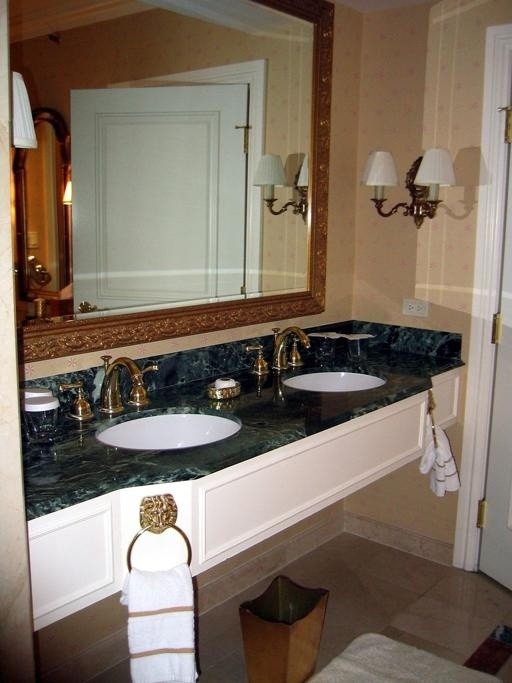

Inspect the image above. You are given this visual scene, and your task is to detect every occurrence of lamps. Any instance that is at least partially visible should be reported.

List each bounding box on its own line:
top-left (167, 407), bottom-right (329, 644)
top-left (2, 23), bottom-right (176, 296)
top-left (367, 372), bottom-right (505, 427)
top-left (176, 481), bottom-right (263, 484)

top-left (252, 153), bottom-right (310, 223)
top-left (10, 71), bottom-right (40, 151)
top-left (360, 147), bottom-right (457, 230)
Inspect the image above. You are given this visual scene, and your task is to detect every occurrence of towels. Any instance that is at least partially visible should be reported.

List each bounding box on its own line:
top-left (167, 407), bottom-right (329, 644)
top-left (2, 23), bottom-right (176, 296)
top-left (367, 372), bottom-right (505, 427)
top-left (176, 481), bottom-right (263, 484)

top-left (418, 425), bottom-right (462, 498)
top-left (119, 562), bottom-right (202, 682)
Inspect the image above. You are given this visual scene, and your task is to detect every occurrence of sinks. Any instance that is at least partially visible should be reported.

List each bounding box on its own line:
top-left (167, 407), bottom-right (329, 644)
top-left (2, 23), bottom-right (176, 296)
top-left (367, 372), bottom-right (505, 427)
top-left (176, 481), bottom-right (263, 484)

top-left (281, 368), bottom-right (388, 393)
top-left (95, 406), bottom-right (242, 450)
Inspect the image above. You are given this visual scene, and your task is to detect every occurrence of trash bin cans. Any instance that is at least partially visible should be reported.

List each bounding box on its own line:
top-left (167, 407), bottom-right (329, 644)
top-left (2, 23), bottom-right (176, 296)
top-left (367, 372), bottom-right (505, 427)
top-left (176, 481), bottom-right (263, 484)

top-left (240, 575), bottom-right (329, 683)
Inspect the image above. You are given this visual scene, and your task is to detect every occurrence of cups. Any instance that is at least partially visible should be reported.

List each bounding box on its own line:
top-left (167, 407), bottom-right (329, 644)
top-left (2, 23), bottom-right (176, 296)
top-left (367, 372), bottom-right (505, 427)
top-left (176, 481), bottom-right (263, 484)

top-left (306, 332), bottom-right (376, 365)
top-left (24, 387), bottom-right (60, 444)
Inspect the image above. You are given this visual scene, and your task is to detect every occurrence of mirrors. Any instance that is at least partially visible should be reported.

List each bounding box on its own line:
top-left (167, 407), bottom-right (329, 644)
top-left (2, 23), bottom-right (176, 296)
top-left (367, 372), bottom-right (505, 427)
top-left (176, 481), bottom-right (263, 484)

top-left (12, 107), bottom-right (72, 306)
top-left (7, 0), bottom-right (337, 367)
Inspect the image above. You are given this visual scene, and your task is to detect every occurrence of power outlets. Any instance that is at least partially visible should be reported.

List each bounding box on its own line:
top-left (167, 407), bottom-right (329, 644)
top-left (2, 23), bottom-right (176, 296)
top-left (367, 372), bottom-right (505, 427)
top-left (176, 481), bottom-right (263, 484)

top-left (402, 297), bottom-right (429, 318)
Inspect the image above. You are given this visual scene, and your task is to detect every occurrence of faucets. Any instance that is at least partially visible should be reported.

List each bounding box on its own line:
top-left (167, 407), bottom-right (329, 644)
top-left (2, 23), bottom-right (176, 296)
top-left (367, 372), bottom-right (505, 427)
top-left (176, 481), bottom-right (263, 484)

top-left (271, 327), bottom-right (311, 368)
top-left (100, 358), bottom-right (152, 413)
top-left (274, 371), bottom-right (292, 408)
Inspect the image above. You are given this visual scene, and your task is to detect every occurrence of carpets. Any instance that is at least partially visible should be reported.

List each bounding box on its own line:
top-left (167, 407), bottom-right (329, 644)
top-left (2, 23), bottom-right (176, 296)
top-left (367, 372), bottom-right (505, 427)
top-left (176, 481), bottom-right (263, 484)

top-left (309, 632), bottom-right (502, 683)
